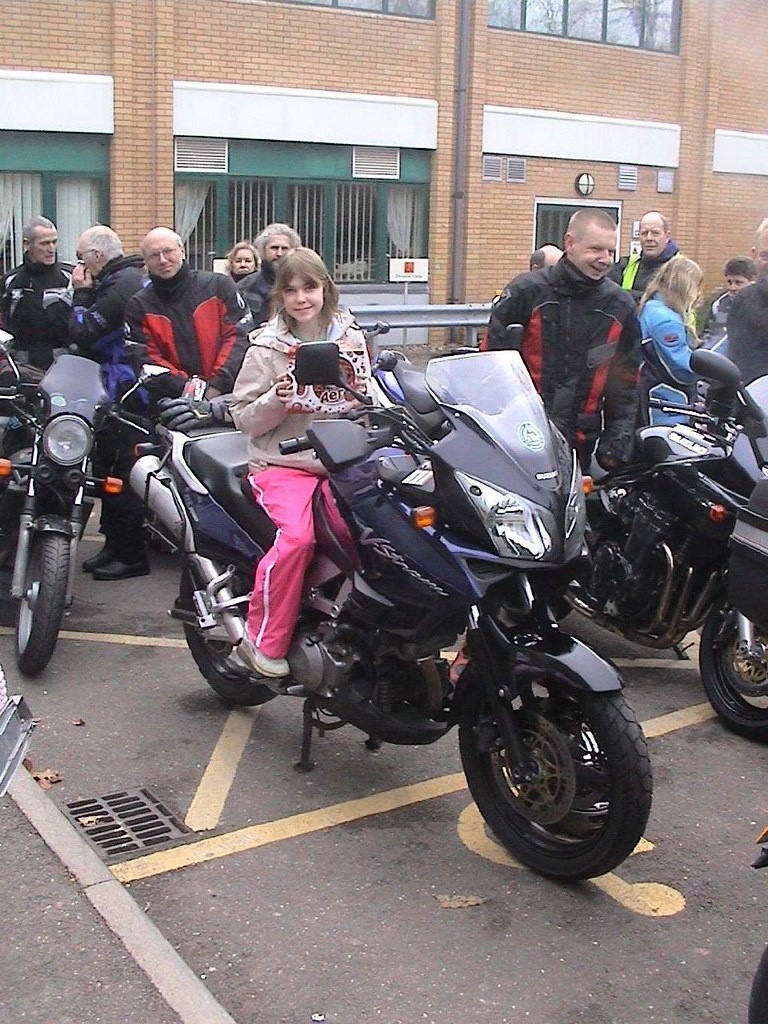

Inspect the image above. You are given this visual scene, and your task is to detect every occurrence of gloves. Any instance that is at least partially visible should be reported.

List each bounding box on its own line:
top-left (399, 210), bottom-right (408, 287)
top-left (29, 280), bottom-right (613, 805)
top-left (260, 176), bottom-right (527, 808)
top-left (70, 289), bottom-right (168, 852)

top-left (159, 395), bottom-right (233, 431)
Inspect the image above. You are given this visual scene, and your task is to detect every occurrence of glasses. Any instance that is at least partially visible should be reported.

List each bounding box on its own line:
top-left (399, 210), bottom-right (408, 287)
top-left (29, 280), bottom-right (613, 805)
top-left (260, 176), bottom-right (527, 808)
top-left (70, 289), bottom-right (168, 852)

top-left (145, 245), bottom-right (181, 262)
top-left (76, 249), bottom-right (104, 264)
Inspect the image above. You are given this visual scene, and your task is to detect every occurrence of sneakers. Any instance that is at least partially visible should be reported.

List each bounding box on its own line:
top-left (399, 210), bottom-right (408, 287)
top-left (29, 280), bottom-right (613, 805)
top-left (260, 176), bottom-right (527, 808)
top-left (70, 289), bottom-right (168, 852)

top-left (240, 622), bottom-right (290, 678)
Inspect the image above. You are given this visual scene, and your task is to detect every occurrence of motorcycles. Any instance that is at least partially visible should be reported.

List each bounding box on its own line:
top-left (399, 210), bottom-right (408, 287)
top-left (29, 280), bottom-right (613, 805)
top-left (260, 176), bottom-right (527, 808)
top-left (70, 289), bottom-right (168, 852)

top-left (554, 348), bottom-right (767, 736)
top-left (1, 329), bottom-right (170, 678)
top-left (131, 325), bottom-right (656, 884)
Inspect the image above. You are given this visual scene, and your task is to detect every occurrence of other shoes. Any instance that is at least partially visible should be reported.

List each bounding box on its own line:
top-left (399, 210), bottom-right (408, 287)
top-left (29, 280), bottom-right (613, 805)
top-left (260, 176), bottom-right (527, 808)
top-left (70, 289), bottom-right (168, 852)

top-left (92, 558), bottom-right (150, 581)
top-left (82, 545), bottom-right (116, 570)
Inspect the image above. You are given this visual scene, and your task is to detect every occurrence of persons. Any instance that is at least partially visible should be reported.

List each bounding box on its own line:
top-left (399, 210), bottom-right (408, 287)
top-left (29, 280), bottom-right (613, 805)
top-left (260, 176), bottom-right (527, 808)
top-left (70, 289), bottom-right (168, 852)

top-left (229, 247), bottom-right (379, 676)
top-left (530, 210), bottom-right (768, 476)
top-left (0, 216), bottom-right (303, 581)
top-left (450, 208), bottom-right (643, 683)
